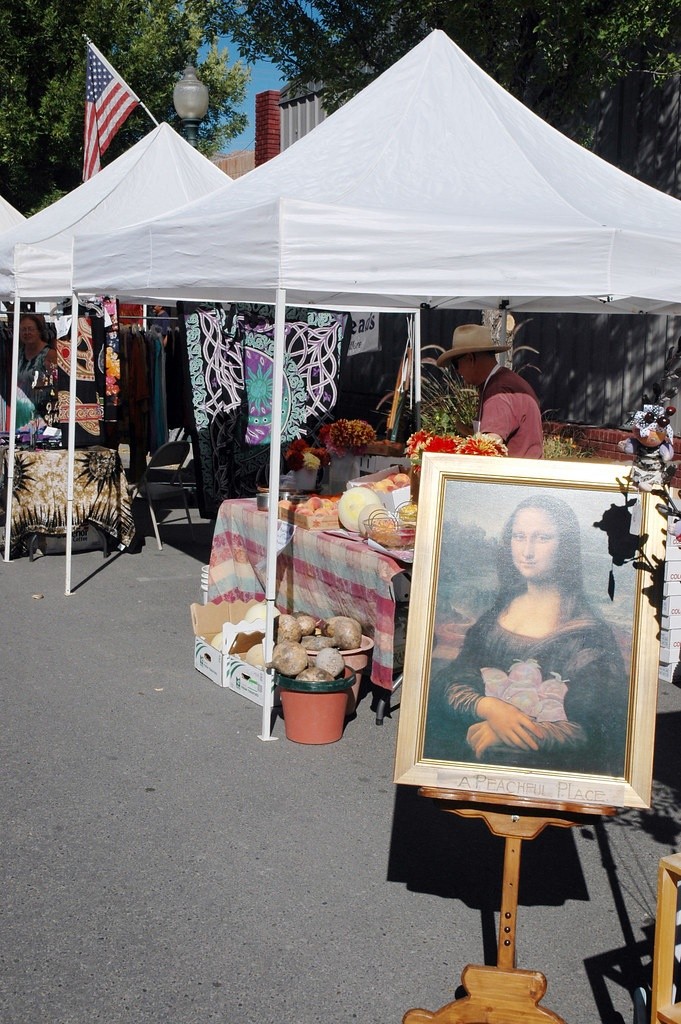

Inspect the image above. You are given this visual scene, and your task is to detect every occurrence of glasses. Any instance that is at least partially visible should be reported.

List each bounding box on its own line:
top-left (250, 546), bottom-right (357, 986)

top-left (449, 354), bottom-right (466, 371)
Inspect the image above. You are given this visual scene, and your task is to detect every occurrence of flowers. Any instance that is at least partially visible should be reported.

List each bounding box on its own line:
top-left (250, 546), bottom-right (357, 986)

top-left (404, 430), bottom-right (508, 477)
top-left (318, 418), bottom-right (377, 459)
top-left (284, 439), bottom-right (330, 472)
top-left (372, 439), bottom-right (402, 455)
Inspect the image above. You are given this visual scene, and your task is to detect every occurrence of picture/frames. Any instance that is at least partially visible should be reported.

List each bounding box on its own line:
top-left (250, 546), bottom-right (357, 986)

top-left (392, 449), bottom-right (670, 808)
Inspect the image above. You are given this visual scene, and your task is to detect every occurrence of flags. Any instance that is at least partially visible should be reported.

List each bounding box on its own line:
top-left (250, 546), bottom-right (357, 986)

top-left (82, 43), bottom-right (138, 183)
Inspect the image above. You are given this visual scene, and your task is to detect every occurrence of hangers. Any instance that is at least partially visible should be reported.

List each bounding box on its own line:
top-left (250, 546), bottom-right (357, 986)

top-left (50, 289), bottom-right (103, 318)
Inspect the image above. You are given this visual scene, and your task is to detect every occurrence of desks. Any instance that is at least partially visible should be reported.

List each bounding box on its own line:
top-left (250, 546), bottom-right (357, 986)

top-left (207, 498), bottom-right (410, 689)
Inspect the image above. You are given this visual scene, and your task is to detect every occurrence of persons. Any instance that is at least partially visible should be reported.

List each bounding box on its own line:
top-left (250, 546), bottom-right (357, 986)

top-left (103, 297), bottom-right (115, 324)
top-left (18, 314), bottom-right (57, 417)
top-left (152, 305), bottom-right (170, 347)
top-left (436, 323), bottom-right (544, 460)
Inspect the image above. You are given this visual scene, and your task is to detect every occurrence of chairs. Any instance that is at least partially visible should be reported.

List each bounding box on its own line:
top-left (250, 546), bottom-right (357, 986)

top-left (128, 440), bottom-right (196, 551)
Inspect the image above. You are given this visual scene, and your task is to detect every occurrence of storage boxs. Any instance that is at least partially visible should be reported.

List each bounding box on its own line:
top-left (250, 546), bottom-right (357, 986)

top-left (358, 455), bottom-right (410, 473)
top-left (278, 506), bottom-right (341, 531)
top-left (346, 464), bottom-right (410, 516)
top-left (190, 599), bottom-right (282, 708)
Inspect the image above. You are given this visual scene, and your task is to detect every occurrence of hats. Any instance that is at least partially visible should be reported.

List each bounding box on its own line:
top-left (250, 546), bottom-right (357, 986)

top-left (434, 324), bottom-right (511, 367)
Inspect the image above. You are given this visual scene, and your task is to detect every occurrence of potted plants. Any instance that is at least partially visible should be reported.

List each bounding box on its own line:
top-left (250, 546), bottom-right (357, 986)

top-left (280, 467), bottom-right (318, 490)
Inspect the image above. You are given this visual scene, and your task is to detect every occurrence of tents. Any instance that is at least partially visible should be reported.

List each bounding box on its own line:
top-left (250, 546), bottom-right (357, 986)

top-left (64, 28), bottom-right (681, 741)
top-left (0, 121), bottom-right (235, 562)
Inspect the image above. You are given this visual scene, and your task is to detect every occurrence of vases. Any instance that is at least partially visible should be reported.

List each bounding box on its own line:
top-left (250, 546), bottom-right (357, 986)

top-left (329, 456), bottom-right (360, 494)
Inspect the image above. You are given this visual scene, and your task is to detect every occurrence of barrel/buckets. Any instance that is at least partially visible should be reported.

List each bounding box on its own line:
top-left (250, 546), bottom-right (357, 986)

top-left (200, 565), bottom-right (210, 605)
top-left (273, 663), bottom-right (357, 745)
top-left (305, 628), bottom-right (376, 716)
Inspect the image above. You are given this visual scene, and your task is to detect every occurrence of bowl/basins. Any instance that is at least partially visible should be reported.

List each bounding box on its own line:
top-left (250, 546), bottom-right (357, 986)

top-left (338, 487), bottom-right (380, 532)
top-left (362, 517), bottom-right (417, 547)
top-left (358, 504), bottom-right (388, 537)
top-left (279, 491), bottom-right (294, 502)
top-left (287, 495), bottom-right (310, 504)
top-left (256, 492), bottom-right (281, 510)
